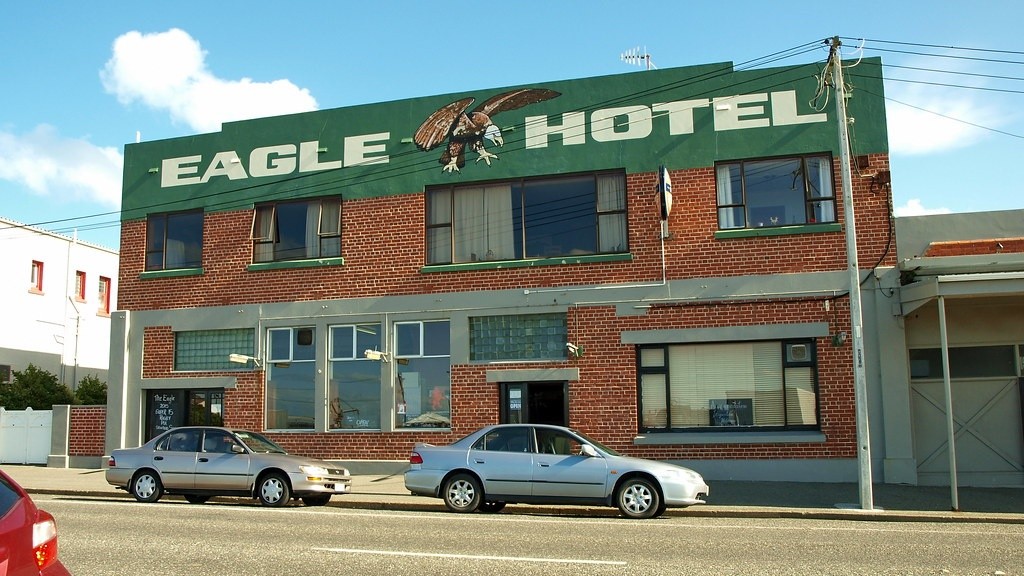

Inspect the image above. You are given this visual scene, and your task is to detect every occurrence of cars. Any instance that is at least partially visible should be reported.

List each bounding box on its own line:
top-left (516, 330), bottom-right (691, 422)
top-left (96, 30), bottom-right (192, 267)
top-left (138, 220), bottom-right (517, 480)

top-left (0, 470), bottom-right (71, 576)
top-left (106, 425), bottom-right (352, 508)
top-left (403, 423), bottom-right (708, 519)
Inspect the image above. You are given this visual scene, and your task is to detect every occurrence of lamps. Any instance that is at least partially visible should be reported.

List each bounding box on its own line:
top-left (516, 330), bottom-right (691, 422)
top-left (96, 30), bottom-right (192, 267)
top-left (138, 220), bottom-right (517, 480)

top-left (566, 342), bottom-right (579, 358)
top-left (229, 353), bottom-right (260, 368)
top-left (365, 349), bottom-right (388, 364)
